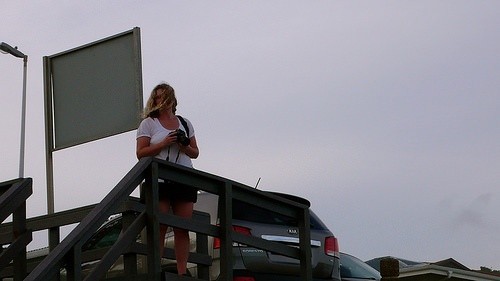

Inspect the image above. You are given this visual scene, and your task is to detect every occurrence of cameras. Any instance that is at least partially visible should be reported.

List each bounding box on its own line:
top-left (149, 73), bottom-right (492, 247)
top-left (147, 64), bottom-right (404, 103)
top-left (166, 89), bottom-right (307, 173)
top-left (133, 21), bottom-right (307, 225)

top-left (168, 129), bottom-right (191, 146)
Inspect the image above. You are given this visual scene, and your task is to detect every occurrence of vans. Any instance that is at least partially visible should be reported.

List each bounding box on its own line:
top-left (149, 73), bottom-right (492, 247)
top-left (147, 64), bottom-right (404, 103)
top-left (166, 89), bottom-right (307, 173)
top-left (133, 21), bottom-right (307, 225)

top-left (61, 191), bottom-right (340, 281)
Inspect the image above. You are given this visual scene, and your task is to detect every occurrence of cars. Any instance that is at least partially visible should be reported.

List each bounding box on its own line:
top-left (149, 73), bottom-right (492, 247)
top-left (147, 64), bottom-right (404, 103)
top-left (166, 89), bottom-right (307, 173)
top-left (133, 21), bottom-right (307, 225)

top-left (341, 251), bottom-right (432, 281)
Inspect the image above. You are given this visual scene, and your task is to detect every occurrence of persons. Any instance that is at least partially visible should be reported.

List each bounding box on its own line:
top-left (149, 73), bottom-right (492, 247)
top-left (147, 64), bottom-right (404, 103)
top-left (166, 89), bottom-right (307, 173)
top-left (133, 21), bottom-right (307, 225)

top-left (135, 84), bottom-right (199, 277)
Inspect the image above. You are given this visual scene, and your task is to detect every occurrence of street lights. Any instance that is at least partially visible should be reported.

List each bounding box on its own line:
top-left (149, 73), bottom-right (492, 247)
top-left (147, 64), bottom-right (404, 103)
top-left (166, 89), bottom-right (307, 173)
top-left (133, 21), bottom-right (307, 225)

top-left (1, 41), bottom-right (28, 178)
